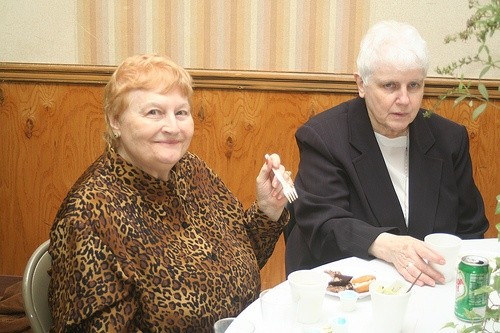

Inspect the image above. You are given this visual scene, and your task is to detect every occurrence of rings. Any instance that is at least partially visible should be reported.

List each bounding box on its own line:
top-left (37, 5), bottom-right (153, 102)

top-left (285, 170), bottom-right (291, 177)
top-left (404, 262), bottom-right (413, 270)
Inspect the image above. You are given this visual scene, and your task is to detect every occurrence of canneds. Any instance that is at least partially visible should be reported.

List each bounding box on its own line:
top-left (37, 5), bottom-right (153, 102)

top-left (454, 255), bottom-right (490, 324)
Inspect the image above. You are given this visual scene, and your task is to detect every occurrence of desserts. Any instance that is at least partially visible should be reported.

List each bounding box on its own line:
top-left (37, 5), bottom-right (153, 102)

top-left (326, 273), bottom-right (354, 293)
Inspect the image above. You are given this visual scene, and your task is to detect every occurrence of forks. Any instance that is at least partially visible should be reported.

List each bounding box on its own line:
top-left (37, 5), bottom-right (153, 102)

top-left (265, 154), bottom-right (298, 204)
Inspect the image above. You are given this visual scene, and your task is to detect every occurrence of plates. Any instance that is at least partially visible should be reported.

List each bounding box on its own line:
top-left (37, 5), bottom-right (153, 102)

top-left (321, 269), bottom-right (379, 298)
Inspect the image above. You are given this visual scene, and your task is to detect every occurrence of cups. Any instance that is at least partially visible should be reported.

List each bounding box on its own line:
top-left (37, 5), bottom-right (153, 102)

top-left (259, 288), bottom-right (301, 333)
top-left (213, 317), bottom-right (256, 333)
top-left (424, 234), bottom-right (465, 282)
top-left (340, 291), bottom-right (359, 313)
top-left (287, 268), bottom-right (332, 321)
top-left (368, 278), bottom-right (412, 330)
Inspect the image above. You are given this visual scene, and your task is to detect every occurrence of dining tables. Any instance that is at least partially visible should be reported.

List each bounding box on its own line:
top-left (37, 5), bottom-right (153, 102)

top-left (223, 239), bottom-right (500, 333)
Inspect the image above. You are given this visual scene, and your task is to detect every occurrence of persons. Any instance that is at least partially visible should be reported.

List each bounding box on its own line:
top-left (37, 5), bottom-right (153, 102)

top-left (47, 55), bottom-right (292, 333)
top-left (293, 21), bottom-right (490, 287)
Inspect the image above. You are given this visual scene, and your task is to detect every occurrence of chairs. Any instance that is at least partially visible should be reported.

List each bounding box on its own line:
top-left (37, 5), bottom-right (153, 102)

top-left (22, 239), bottom-right (52, 333)
